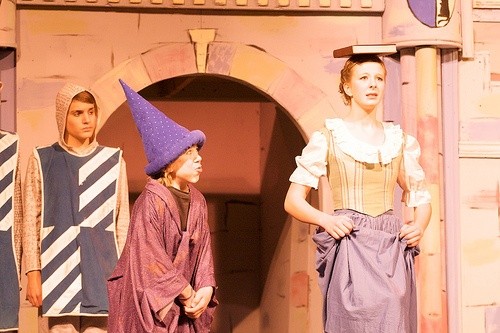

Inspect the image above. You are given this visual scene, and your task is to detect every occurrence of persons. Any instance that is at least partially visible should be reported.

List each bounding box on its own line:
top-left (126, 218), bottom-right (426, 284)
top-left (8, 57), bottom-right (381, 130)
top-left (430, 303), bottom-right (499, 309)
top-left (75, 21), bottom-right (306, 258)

top-left (21, 84), bottom-right (130, 333)
top-left (108, 79), bottom-right (219, 333)
top-left (284, 53), bottom-right (432, 333)
top-left (0, 128), bottom-right (23, 333)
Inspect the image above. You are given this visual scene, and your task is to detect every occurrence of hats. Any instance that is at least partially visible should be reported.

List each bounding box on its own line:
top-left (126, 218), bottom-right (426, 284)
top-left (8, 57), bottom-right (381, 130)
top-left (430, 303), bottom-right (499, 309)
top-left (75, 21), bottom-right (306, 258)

top-left (119, 77), bottom-right (205, 176)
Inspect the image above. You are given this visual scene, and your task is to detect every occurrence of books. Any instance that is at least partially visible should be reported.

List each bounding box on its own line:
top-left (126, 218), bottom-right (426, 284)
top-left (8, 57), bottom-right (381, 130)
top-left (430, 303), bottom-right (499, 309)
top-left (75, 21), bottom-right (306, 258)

top-left (333, 44), bottom-right (397, 58)
